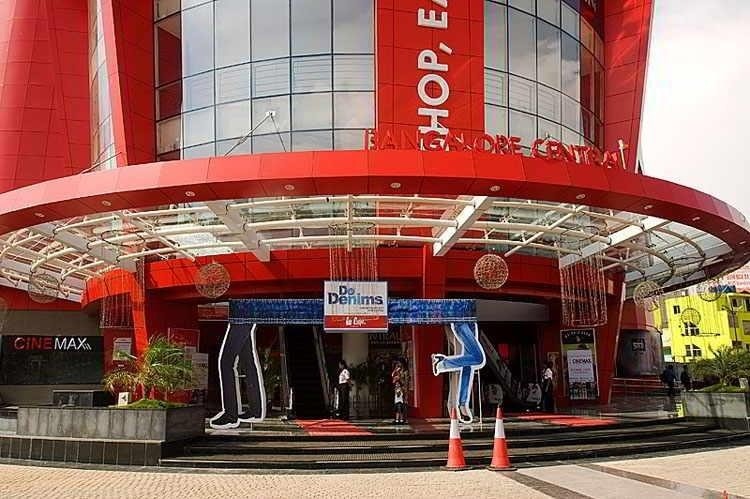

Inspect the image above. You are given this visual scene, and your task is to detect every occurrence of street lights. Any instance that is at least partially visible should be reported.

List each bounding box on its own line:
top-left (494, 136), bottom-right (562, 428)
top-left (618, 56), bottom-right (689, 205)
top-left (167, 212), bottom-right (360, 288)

top-left (721, 299), bottom-right (744, 353)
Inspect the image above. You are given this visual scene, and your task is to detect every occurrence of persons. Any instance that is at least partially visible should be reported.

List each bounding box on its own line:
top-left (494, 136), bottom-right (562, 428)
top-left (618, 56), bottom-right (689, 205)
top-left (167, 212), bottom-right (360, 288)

top-left (334, 359), bottom-right (354, 419)
top-left (539, 361), bottom-right (557, 412)
top-left (394, 380), bottom-right (406, 425)
top-left (680, 363), bottom-right (692, 392)
top-left (208, 323), bottom-right (267, 430)
top-left (664, 364), bottom-right (677, 397)
top-left (431, 320), bottom-right (488, 426)
top-left (391, 357), bottom-right (411, 423)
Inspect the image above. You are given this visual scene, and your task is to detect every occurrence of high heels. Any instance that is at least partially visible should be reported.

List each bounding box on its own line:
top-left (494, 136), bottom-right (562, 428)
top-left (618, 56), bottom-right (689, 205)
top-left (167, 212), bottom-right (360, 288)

top-left (458, 405), bottom-right (471, 423)
top-left (432, 354), bottom-right (445, 376)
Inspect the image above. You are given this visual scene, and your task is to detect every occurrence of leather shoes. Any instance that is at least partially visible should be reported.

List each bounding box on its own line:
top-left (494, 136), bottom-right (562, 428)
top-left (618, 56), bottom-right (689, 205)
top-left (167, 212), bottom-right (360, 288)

top-left (238, 409), bottom-right (262, 420)
top-left (210, 412), bottom-right (238, 425)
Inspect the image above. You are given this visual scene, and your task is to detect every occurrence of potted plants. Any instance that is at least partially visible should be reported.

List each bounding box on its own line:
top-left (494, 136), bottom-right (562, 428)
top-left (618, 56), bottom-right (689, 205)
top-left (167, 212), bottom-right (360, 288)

top-left (688, 342), bottom-right (749, 390)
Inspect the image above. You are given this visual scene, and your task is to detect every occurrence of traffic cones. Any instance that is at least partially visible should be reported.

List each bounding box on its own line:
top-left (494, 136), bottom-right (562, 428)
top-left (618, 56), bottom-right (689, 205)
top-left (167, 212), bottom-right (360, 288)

top-left (485, 403), bottom-right (518, 472)
top-left (439, 408), bottom-right (474, 471)
